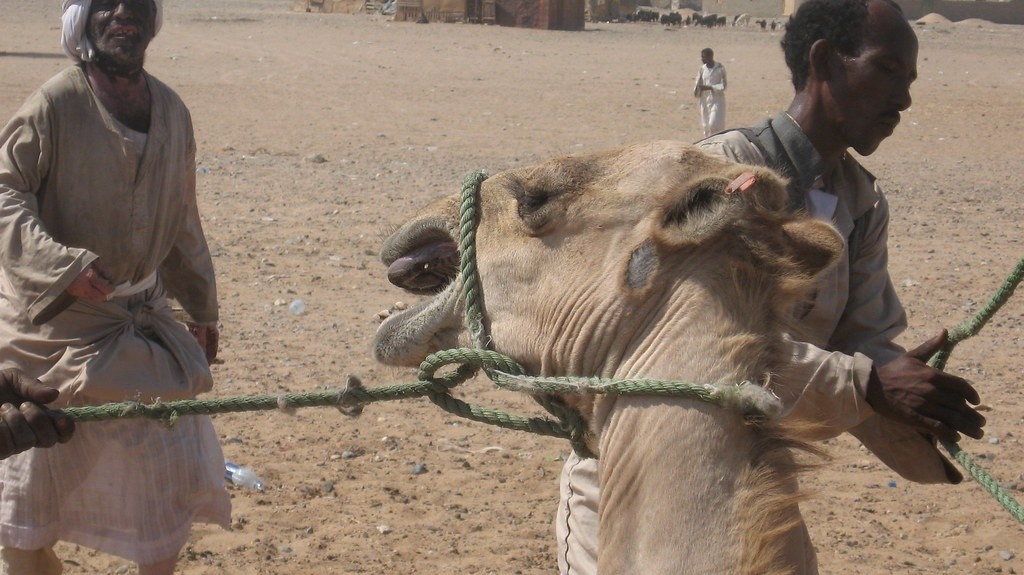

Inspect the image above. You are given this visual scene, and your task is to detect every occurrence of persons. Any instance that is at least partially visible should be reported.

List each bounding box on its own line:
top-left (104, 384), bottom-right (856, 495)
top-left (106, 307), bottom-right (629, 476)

top-left (0, 0), bottom-right (233, 575)
top-left (0, 367), bottom-right (76, 460)
top-left (695, 48), bottom-right (727, 138)
top-left (556, 0), bottom-right (987, 575)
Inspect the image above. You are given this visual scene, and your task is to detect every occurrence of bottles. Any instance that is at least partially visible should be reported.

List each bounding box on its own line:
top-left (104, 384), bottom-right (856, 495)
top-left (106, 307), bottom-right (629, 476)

top-left (222, 459), bottom-right (265, 491)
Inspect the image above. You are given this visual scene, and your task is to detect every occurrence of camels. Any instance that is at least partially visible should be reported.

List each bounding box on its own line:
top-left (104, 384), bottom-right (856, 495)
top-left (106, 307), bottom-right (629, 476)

top-left (372, 139), bottom-right (851, 575)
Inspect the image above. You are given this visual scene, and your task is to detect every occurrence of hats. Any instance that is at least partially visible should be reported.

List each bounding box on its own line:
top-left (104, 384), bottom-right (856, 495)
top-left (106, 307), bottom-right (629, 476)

top-left (61, 0), bottom-right (164, 63)
